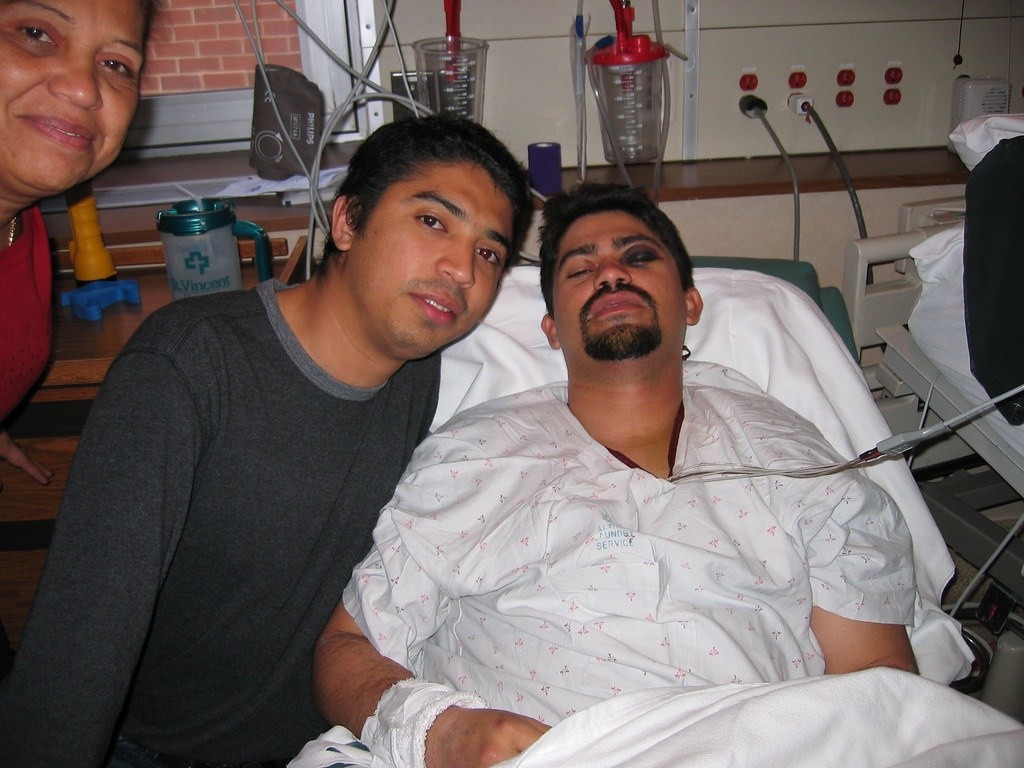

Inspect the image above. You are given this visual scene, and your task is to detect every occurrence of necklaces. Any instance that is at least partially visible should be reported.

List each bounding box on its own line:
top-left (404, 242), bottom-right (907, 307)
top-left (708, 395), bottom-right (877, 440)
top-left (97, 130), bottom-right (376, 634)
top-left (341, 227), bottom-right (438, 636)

top-left (7, 215), bottom-right (18, 247)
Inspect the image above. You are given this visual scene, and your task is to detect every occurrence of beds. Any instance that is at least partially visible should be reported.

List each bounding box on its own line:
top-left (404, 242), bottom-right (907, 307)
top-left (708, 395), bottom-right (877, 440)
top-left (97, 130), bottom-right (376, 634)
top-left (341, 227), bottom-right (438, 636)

top-left (850, 196), bottom-right (1024, 608)
top-left (299, 254), bottom-right (1024, 768)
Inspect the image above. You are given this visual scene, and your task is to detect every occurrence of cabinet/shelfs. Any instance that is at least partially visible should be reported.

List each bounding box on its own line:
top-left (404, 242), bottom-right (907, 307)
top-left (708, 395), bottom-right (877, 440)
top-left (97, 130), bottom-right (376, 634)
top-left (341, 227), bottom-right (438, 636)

top-left (0, 237), bottom-right (291, 648)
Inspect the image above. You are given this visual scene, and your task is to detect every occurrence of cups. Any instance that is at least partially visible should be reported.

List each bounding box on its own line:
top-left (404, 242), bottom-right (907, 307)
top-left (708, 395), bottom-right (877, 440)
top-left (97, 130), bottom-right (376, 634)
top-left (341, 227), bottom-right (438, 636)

top-left (413, 36), bottom-right (488, 122)
top-left (589, 35), bottom-right (663, 164)
top-left (155, 198), bottom-right (274, 303)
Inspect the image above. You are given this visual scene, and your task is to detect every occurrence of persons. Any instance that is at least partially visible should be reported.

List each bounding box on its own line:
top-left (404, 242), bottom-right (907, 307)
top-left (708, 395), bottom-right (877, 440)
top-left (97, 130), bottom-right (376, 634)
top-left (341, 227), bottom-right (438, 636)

top-left (311, 181), bottom-right (920, 768)
top-left (0, 110), bottom-right (535, 767)
top-left (0, 0), bottom-right (159, 488)
